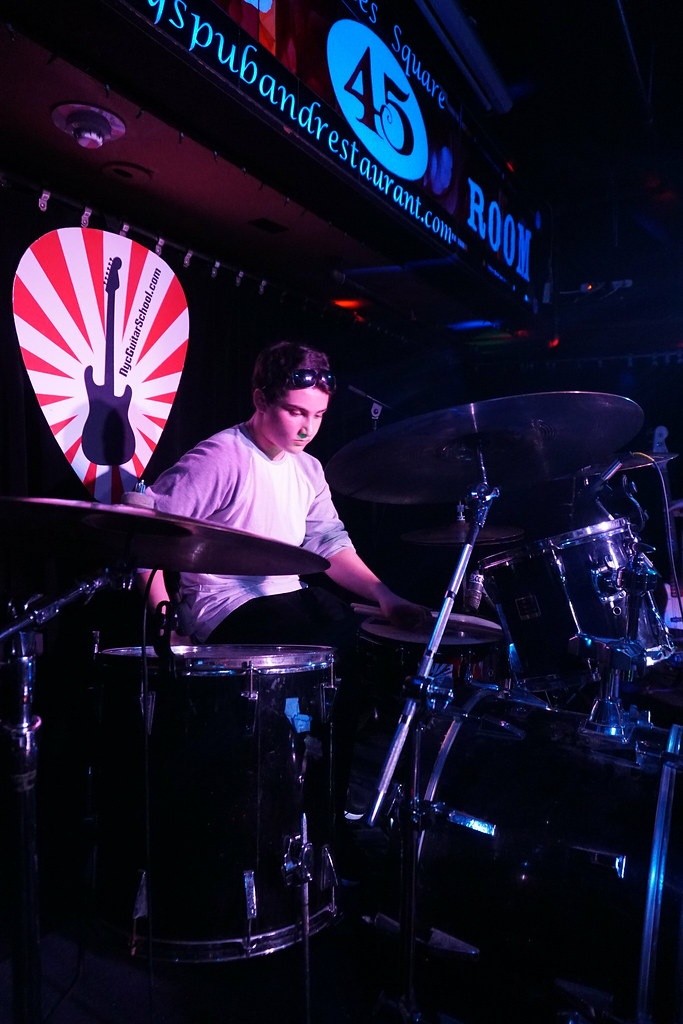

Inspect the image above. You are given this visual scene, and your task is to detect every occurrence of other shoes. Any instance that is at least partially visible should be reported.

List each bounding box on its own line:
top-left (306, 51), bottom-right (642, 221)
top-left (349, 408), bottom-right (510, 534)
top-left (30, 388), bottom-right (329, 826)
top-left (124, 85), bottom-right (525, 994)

top-left (344, 803), bottom-right (368, 820)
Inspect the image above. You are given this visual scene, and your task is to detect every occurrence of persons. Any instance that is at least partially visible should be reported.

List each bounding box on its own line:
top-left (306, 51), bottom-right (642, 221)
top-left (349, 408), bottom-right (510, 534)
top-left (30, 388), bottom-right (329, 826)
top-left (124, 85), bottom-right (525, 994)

top-left (130, 343), bottom-right (434, 886)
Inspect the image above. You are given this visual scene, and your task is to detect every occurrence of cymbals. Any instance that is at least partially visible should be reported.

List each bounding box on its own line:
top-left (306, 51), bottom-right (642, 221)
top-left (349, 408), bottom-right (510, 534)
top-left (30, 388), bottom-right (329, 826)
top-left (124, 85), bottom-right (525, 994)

top-left (0, 494), bottom-right (330, 578)
top-left (326, 388), bottom-right (643, 509)
top-left (399, 517), bottom-right (527, 547)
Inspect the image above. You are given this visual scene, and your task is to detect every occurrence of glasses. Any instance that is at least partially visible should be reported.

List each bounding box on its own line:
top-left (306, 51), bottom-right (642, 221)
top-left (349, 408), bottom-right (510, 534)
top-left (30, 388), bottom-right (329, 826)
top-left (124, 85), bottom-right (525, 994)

top-left (291, 367), bottom-right (336, 395)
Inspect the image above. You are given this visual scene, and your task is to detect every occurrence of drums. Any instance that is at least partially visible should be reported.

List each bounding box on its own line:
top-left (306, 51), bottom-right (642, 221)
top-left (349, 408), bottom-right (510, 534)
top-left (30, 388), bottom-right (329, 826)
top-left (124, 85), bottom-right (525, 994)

top-left (388, 672), bottom-right (683, 1024)
top-left (480, 516), bottom-right (674, 691)
top-left (99, 642), bottom-right (339, 968)
top-left (355, 611), bottom-right (510, 686)
